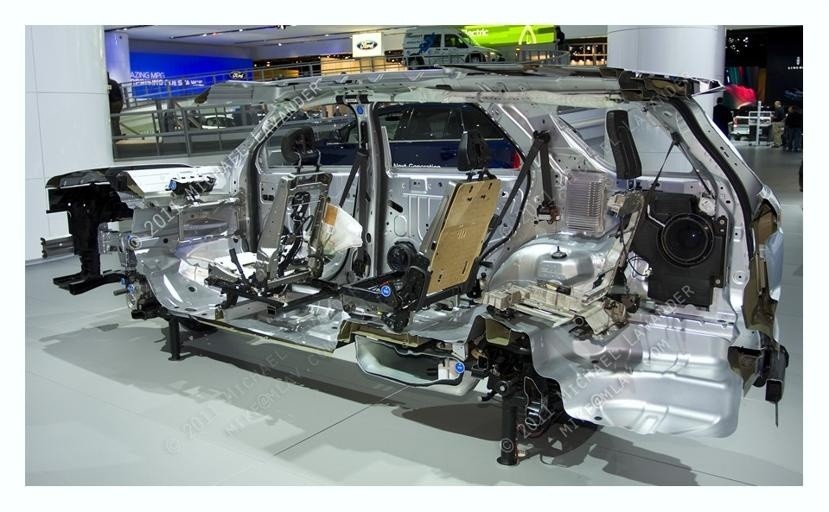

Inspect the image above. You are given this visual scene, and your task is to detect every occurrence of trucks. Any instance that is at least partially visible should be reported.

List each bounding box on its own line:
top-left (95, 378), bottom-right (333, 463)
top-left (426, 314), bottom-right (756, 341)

top-left (401, 28), bottom-right (507, 69)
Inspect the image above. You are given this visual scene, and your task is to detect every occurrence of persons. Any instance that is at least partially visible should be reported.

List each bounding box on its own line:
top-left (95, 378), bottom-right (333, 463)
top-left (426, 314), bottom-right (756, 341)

top-left (770, 100), bottom-right (786, 148)
top-left (713, 96), bottom-right (733, 138)
top-left (107, 71), bottom-right (124, 136)
top-left (785, 106), bottom-right (802, 153)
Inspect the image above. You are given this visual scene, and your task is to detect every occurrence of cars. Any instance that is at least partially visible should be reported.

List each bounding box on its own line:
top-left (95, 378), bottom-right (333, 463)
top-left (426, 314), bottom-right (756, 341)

top-left (299, 103), bottom-right (526, 172)
top-left (192, 103), bottom-right (328, 131)
